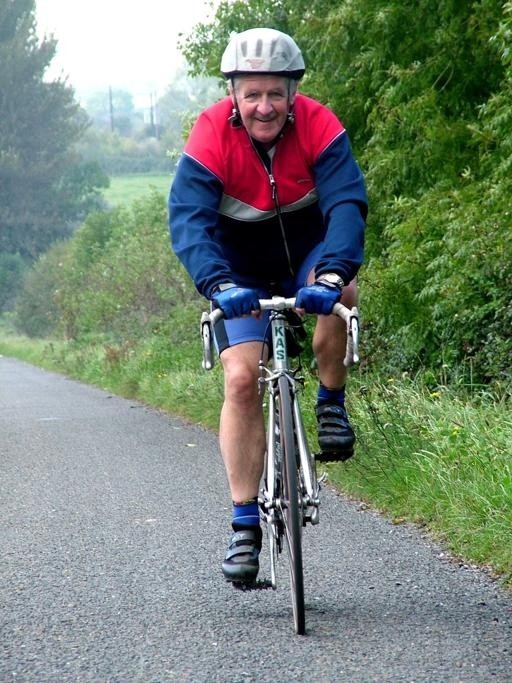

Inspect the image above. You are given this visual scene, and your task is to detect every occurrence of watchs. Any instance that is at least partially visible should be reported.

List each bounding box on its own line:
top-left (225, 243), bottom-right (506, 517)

top-left (316, 272), bottom-right (345, 289)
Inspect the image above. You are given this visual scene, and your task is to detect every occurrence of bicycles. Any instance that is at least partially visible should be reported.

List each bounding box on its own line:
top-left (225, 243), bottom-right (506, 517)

top-left (178, 293), bottom-right (361, 639)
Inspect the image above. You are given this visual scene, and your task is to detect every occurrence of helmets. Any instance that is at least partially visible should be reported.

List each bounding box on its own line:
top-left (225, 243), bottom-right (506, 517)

top-left (220, 27), bottom-right (306, 80)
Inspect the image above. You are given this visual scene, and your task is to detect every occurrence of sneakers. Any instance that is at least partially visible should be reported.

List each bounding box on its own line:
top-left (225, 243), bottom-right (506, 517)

top-left (220, 522), bottom-right (264, 581)
top-left (316, 404), bottom-right (356, 451)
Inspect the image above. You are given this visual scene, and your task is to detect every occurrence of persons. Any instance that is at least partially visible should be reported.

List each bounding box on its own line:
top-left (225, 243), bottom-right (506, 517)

top-left (169, 27), bottom-right (368, 580)
